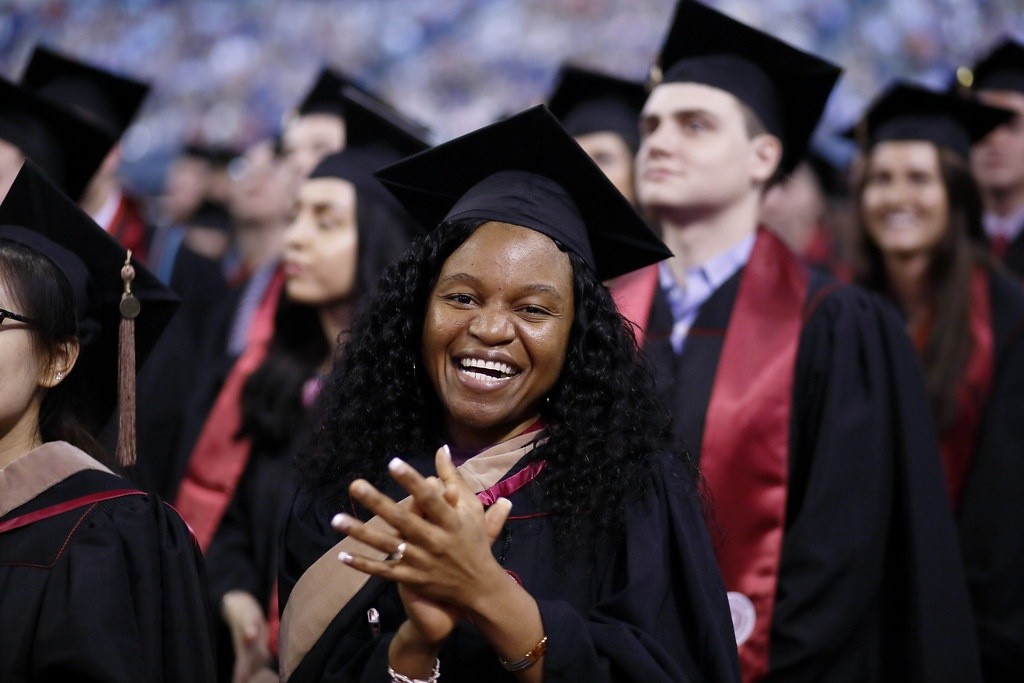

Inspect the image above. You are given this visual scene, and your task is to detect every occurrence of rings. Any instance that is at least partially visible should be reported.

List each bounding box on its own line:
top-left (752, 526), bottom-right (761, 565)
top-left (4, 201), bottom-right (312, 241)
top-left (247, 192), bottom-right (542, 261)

top-left (393, 543), bottom-right (406, 560)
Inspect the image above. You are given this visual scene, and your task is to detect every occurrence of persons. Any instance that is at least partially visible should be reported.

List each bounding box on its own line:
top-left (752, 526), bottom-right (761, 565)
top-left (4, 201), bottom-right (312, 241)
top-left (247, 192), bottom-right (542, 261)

top-left (0, 0), bottom-right (1024, 683)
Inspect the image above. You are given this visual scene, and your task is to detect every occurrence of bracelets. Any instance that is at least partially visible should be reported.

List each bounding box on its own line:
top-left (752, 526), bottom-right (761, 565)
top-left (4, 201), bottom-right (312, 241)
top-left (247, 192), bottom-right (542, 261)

top-left (497, 635), bottom-right (548, 673)
top-left (387, 657), bottom-right (440, 683)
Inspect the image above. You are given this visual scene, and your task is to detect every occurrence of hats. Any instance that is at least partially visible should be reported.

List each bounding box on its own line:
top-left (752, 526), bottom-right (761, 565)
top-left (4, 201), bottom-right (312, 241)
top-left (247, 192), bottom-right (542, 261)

top-left (370, 103), bottom-right (677, 285)
top-left (651, 1), bottom-right (844, 183)
top-left (838, 76), bottom-right (1015, 162)
top-left (0, 45), bottom-right (150, 198)
top-left (299, 66), bottom-right (431, 204)
top-left (552, 64), bottom-right (648, 146)
top-left (960, 36), bottom-right (1024, 94)
top-left (0, 158), bottom-right (182, 467)
